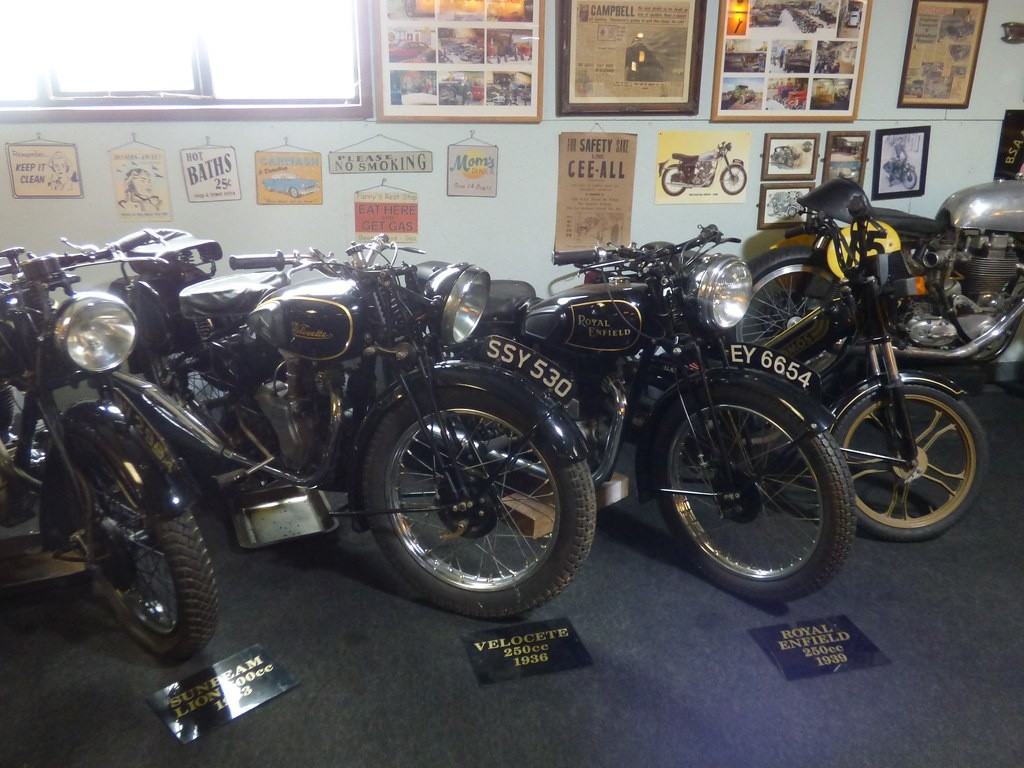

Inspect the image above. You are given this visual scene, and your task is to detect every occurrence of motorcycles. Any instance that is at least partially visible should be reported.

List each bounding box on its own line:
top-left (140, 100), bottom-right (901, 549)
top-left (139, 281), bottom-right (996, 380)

top-left (729, 178), bottom-right (1024, 392)
top-left (1, 177), bottom-right (991, 673)
top-left (883, 157), bottom-right (918, 189)
top-left (786, 6), bottom-right (824, 34)
top-left (657, 141), bottom-right (747, 196)
top-left (772, 198), bottom-right (798, 217)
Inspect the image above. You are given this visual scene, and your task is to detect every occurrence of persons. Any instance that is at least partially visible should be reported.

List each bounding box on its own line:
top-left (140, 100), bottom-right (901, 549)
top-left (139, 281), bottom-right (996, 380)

top-left (889, 137), bottom-right (907, 186)
top-left (785, 77), bottom-right (793, 89)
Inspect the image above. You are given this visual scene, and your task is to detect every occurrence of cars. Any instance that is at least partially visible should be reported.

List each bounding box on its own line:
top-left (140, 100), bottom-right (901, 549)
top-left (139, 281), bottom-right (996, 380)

top-left (836, 141), bottom-right (860, 155)
top-left (514, 85), bottom-right (531, 95)
top-left (729, 85), bottom-right (755, 104)
top-left (770, 145), bottom-right (802, 169)
top-left (389, 39), bottom-right (436, 64)
top-left (847, 11), bottom-right (860, 27)
top-left (808, 7), bottom-right (836, 23)
top-left (402, 0), bottom-right (533, 22)
top-left (447, 43), bottom-right (481, 63)
top-left (749, 8), bottom-right (782, 28)
